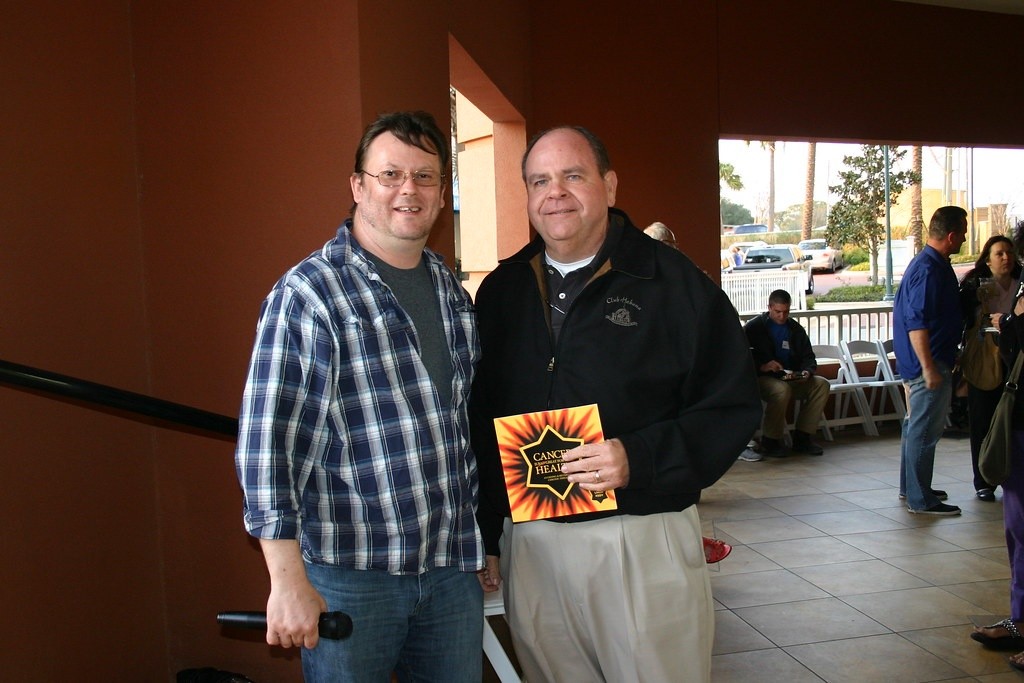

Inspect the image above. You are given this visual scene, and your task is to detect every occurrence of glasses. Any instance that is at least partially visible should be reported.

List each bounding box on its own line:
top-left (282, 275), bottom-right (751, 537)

top-left (662, 238), bottom-right (680, 248)
top-left (361, 168), bottom-right (445, 187)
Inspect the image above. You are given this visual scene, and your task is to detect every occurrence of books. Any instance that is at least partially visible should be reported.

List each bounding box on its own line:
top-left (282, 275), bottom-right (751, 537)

top-left (493, 403), bottom-right (618, 524)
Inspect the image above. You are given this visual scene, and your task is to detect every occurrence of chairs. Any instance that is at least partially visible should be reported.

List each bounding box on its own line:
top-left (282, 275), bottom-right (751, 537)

top-left (755, 338), bottom-right (951, 445)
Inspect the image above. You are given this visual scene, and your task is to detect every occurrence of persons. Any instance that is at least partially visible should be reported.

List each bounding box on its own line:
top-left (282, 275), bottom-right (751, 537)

top-left (702, 537), bottom-right (732, 564)
top-left (952, 235), bottom-right (1024, 503)
top-left (970, 221), bottom-right (1024, 671)
top-left (236, 110), bottom-right (486, 683)
top-left (467, 127), bottom-right (763, 683)
top-left (892, 206), bottom-right (967, 515)
top-left (742, 290), bottom-right (830, 457)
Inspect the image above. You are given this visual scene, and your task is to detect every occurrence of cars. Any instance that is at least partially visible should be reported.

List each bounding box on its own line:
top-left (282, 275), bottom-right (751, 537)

top-left (798, 238), bottom-right (844, 273)
top-left (720, 240), bottom-right (814, 294)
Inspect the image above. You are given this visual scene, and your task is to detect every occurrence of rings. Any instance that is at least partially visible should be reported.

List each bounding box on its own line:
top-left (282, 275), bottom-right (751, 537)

top-left (596, 472), bottom-right (601, 483)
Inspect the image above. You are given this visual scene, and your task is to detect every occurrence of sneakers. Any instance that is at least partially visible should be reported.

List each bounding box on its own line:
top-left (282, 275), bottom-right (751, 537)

top-left (793, 430), bottom-right (823, 454)
top-left (737, 449), bottom-right (762, 462)
top-left (746, 441), bottom-right (757, 448)
top-left (761, 435), bottom-right (789, 457)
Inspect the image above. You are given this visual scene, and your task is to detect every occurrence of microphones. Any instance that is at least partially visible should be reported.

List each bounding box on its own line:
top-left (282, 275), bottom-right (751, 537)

top-left (217, 610), bottom-right (353, 641)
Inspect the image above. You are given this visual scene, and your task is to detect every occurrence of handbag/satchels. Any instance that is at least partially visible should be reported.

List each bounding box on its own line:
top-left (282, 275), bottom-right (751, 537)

top-left (960, 313), bottom-right (1002, 389)
top-left (978, 382), bottom-right (1019, 487)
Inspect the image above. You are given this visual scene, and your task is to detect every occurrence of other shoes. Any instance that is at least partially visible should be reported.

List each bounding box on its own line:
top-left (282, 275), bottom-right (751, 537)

top-left (976, 489), bottom-right (995, 500)
top-left (899, 490), bottom-right (947, 499)
top-left (908, 503), bottom-right (961, 515)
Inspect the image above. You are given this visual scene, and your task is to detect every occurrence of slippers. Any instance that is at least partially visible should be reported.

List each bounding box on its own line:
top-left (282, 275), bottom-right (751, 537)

top-left (970, 618), bottom-right (1024, 649)
top-left (1010, 649), bottom-right (1024, 669)
top-left (702, 536), bottom-right (732, 564)
